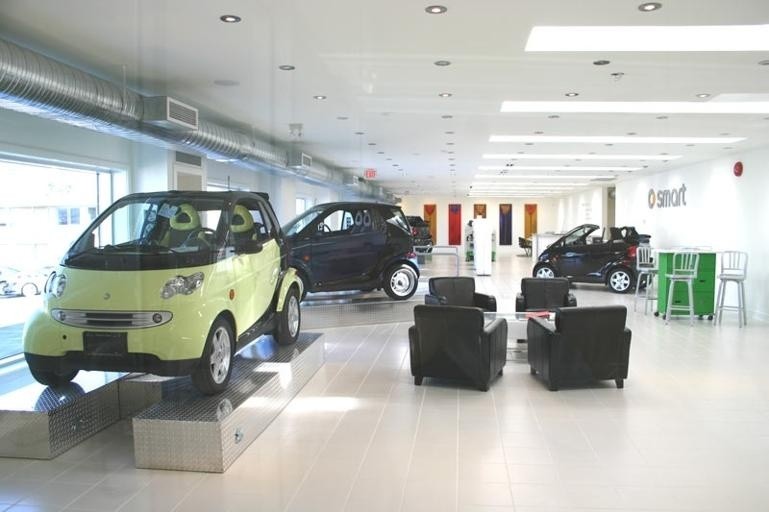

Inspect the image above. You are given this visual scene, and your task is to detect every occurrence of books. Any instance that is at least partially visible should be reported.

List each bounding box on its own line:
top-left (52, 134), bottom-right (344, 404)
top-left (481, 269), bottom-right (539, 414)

top-left (548, 312), bottom-right (556, 321)
top-left (525, 310), bottom-right (550, 319)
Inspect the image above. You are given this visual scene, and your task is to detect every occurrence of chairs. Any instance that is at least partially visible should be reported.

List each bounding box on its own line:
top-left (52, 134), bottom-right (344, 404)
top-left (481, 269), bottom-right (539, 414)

top-left (633, 246), bottom-right (750, 329)
top-left (519, 236), bottom-right (532, 257)
top-left (351, 210), bottom-right (375, 232)
top-left (409, 276), bottom-right (632, 391)
top-left (153, 204), bottom-right (260, 247)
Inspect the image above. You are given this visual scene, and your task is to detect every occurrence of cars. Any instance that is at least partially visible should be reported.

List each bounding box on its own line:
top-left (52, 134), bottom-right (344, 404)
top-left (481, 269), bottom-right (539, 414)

top-left (531, 220), bottom-right (654, 293)
top-left (1, 266), bottom-right (55, 297)
top-left (18, 186), bottom-right (300, 392)
top-left (278, 200), bottom-right (422, 304)
top-left (404, 216), bottom-right (432, 254)
